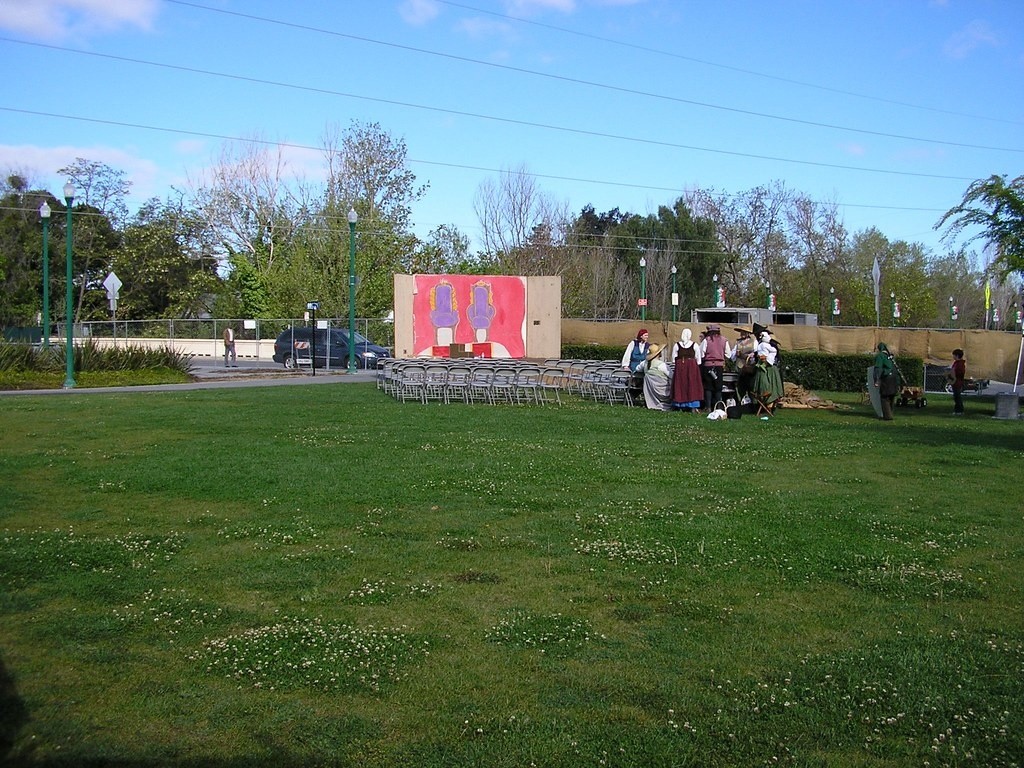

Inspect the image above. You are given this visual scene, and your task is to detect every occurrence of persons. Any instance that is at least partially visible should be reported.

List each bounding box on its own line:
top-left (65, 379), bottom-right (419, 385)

top-left (750, 321), bottom-right (784, 412)
top-left (222, 322), bottom-right (239, 367)
top-left (671, 326), bottom-right (704, 414)
top-left (635, 344), bottom-right (674, 411)
top-left (873, 341), bottom-right (900, 421)
top-left (730, 325), bottom-right (759, 403)
top-left (942, 349), bottom-right (965, 416)
top-left (699, 324), bottom-right (732, 412)
top-left (622, 328), bottom-right (651, 373)
top-left (755, 332), bottom-right (777, 372)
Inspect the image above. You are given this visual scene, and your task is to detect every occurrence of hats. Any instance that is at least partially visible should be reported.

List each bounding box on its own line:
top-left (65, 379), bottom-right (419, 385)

top-left (706, 325), bottom-right (721, 331)
top-left (751, 322), bottom-right (768, 334)
top-left (734, 325), bottom-right (753, 335)
top-left (646, 344), bottom-right (667, 362)
top-left (637, 329), bottom-right (648, 338)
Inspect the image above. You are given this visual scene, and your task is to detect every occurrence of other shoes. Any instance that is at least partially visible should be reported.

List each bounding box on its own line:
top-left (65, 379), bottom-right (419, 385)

top-left (878, 417), bottom-right (893, 421)
top-left (760, 365), bottom-right (768, 373)
top-left (692, 409), bottom-right (701, 413)
top-left (703, 407), bottom-right (711, 412)
top-left (754, 364), bottom-right (760, 369)
top-left (951, 410), bottom-right (962, 415)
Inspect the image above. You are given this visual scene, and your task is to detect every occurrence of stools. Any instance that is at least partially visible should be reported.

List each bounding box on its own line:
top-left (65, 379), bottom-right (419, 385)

top-left (750, 391), bottom-right (774, 417)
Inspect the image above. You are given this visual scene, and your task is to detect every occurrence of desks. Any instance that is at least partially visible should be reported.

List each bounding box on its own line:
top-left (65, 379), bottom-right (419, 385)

top-left (722, 372), bottom-right (739, 382)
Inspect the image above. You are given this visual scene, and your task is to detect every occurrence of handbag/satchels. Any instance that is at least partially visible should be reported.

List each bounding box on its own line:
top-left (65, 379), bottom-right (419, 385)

top-left (727, 394), bottom-right (762, 419)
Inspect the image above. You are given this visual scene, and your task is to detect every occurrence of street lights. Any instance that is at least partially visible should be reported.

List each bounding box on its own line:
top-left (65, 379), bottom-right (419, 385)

top-left (765, 280), bottom-right (771, 309)
top-left (1014, 302), bottom-right (1018, 331)
top-left (712, 273), bottom-right (718, 308)
top-left (890, 291), bottom-right (896, 327)
top-left (990, 299), bottom-right (994, 330)
top-left (949, 295), bottom-right (954, 328)
top-left (830, 287), bottom-right (835, 326)
top-left (61, 181), bottom-right (78, 388)
top-left (348, 207), bottom-right (359, 374)
top-left (671, 265), bottom-right (678, 322)
top-left (639, 256), bottom-right (647, 320)
top-left (39, 200), bottom-right (52, 351)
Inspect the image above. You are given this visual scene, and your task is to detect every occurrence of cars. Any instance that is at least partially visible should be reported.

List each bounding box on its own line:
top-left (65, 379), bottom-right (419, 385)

top-left (924, 361), bottom-right (972, 393)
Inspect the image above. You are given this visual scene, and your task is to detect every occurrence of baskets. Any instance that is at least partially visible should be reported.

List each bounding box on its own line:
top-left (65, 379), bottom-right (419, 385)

top-left (707, 401), bottom-right (728, 422)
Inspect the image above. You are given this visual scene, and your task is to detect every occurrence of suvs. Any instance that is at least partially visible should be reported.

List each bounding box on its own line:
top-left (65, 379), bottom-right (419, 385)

top-left (272, 326), bottom-right (390, 370)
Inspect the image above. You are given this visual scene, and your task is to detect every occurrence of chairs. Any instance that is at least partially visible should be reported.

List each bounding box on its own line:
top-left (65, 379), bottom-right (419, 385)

top-left (376, 357), bottom-right (676, 408)
